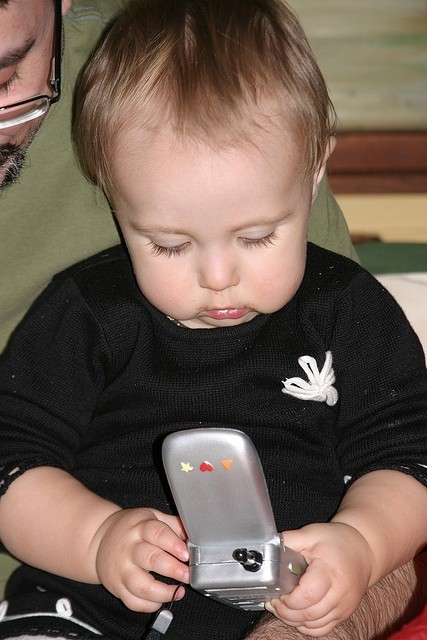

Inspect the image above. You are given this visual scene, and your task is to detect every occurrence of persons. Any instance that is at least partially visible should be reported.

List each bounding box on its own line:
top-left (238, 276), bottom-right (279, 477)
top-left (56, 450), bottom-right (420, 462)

top-left (0, 0), bottom-right (427, 640)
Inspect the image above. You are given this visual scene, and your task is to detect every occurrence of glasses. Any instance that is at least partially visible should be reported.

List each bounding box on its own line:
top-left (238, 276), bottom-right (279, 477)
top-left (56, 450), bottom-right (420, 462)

top-left (0, 0), bottom-right (61, 128)
